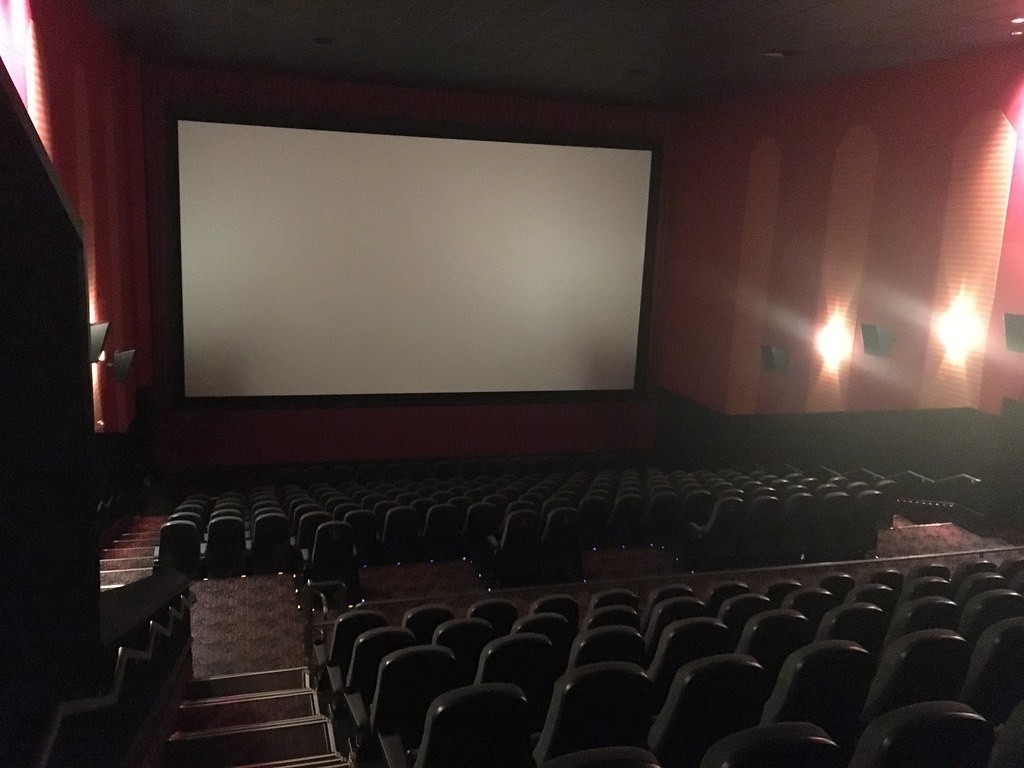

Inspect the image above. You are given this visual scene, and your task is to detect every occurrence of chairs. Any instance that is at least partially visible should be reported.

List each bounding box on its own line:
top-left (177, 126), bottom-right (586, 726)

top-left (36, 438), bottom-right (1024, 768)
